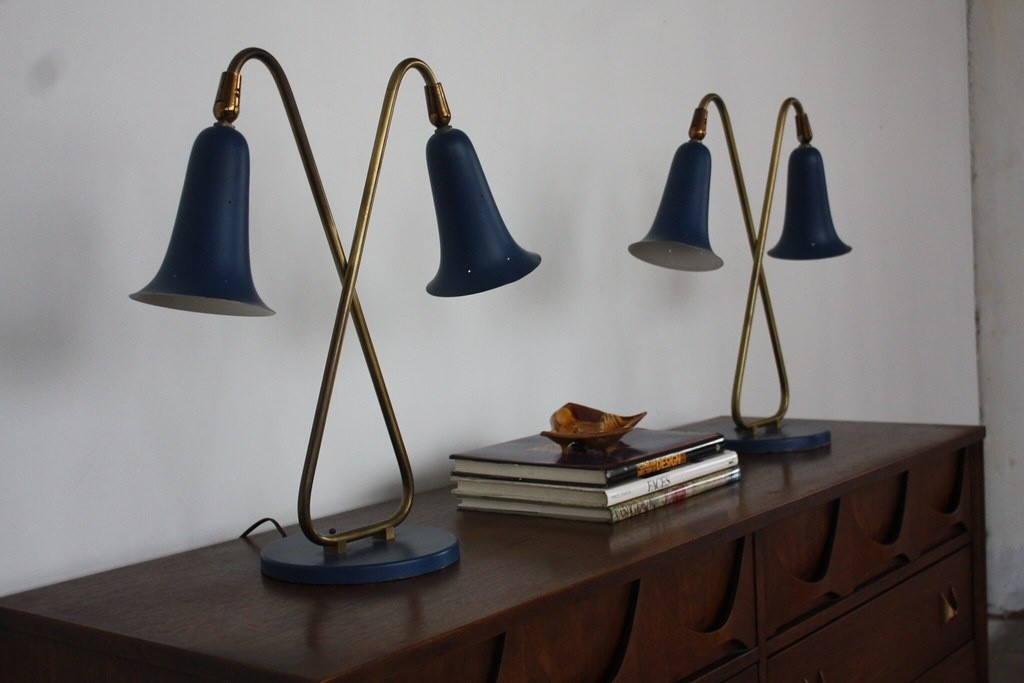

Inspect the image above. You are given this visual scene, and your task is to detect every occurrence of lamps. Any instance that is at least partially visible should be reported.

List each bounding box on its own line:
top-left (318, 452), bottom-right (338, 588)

top-left (129, 48), bottom-right (542, 590)
top-left (628, 93), bottom-right (852, 457)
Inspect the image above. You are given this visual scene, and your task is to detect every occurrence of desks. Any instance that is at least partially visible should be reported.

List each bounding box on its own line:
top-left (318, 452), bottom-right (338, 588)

top-left (0, 417), bottom-right (990, 683)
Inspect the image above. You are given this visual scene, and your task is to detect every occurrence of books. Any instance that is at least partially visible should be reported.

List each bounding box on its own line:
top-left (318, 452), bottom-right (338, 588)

top-left (447, 426), bottom-right (743, 525)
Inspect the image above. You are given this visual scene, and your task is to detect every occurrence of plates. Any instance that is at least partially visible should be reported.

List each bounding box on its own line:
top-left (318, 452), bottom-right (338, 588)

top-left (541, 402), bottom-right (647, 453)
